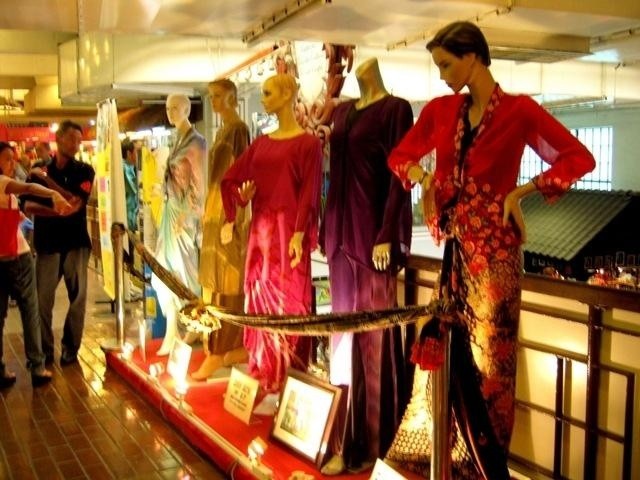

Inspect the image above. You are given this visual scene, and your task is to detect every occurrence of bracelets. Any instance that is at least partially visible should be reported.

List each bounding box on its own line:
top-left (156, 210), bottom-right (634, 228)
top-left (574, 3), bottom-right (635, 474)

top-left (418, 170), bottom-right (427, 187)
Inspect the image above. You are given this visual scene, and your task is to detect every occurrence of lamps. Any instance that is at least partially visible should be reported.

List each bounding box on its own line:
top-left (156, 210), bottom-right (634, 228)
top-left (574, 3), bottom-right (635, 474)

top-left (246, 437), bottom-right (268, 464)
top-left (147, 361), bottom-right (164, 383)
top-left (121, 337), bottom-right (139, 360)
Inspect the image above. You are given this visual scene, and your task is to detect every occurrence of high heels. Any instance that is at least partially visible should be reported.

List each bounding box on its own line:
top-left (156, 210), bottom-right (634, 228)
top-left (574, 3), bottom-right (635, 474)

top-left (31, 368), bottom-right (51, 387)
top-left (0, 368), bottom-right (16, 389)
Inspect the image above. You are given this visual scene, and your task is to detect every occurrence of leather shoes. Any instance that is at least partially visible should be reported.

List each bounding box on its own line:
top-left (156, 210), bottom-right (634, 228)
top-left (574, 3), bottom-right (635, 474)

top-left (27, 355), bottom-right (54, 368)
top-left (61, 351), bottom-right (77, 365)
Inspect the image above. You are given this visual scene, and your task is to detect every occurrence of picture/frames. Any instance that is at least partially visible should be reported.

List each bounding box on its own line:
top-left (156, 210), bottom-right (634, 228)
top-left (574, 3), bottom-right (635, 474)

top-left (270, 367), bottom-right (343, 470)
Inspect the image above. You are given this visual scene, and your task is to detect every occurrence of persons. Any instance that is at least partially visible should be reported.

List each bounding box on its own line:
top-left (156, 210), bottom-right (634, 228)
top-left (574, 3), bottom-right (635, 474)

top-left (8, 139), bottom-right (55, 308)
top-left (387, 19), bottom-right (597, 479)
top-left (16, 119), bottom-right (96, 370)
top-left (0, 141), bottom-right (73, 390)
top-left (219, 73), bottom-right (324, 420)
top-left (122, 136), bottom-right (145, 304)
top-left (190, 77), bottom-right (251, 382)
top-left (148, 91), bottom-right (208, 358)
top-left (317, 55), bottom-right (415, 477)
